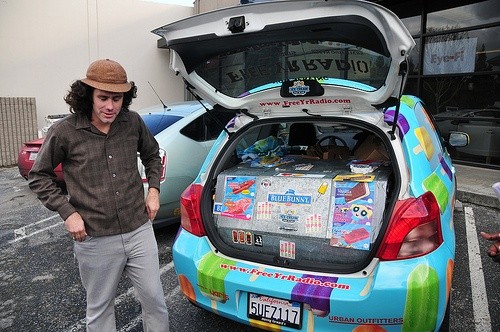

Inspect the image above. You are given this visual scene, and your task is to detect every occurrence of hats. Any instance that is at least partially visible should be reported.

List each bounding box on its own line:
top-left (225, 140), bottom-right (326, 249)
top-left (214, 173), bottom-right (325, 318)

top-left (81, 59), bottom-right (131, 93)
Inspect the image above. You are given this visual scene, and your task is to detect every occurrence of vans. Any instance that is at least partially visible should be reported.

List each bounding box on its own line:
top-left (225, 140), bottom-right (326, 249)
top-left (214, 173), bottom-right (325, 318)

top-left (149, 0), bottom-right (471, 331)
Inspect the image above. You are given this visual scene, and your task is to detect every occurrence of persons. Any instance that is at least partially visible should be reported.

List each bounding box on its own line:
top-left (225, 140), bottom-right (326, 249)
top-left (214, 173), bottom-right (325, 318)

top-left (480, 181), bottom-right (500, 263)
top-left (288, 122), bottom-right (318, 148)
top-left (27, 58), bottom-right (170, 332)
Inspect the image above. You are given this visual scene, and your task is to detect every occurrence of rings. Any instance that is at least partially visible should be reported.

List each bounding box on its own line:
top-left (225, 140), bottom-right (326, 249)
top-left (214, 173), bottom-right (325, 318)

top-left (71, 235), bottom-right (76, 240)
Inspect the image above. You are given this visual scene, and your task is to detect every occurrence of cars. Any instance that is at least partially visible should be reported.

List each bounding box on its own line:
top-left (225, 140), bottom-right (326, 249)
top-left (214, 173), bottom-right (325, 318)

top-left (17, 133), bottom-right (64, 182)
top-left (433, 108), bottom-right (500, 158)
top-left (139, 80), bottom-right (236, 231)
